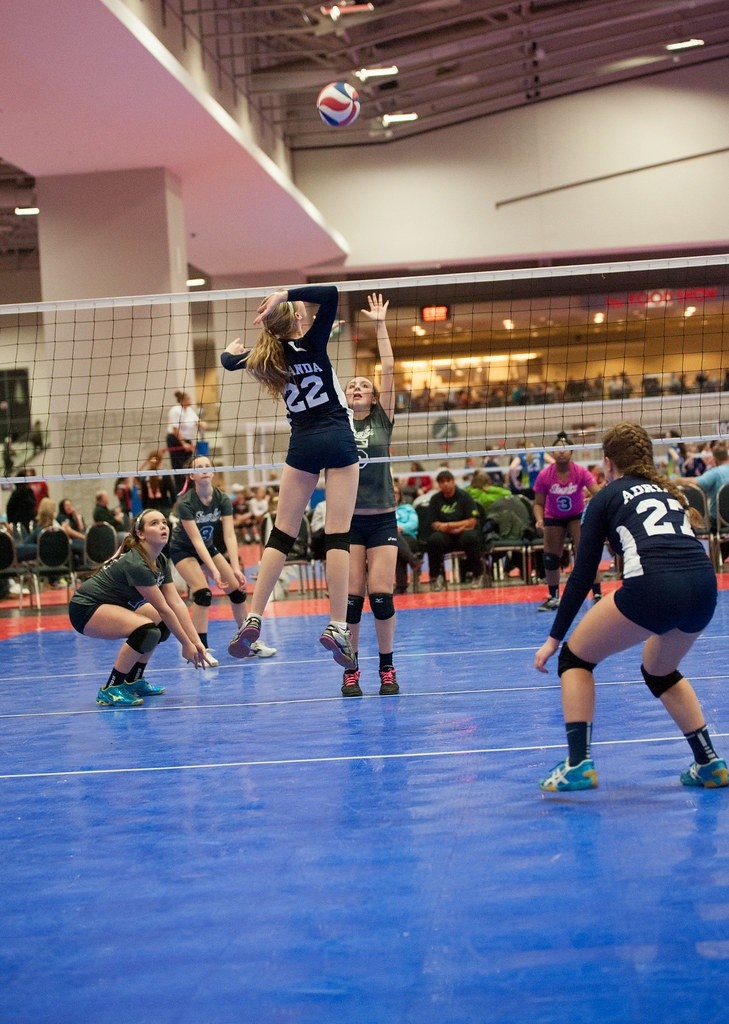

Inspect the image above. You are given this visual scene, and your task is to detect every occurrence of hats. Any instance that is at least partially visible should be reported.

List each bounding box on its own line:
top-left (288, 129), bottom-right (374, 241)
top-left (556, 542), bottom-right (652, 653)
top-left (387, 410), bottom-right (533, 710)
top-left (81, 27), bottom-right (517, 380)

top-left (232, 483), bottom-right (244, 492)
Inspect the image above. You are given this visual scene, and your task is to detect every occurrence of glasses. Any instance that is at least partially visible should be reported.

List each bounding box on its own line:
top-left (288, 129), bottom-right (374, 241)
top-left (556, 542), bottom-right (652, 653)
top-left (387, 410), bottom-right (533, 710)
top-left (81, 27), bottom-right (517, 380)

top-left (394, 492), bottom-right (401, 495)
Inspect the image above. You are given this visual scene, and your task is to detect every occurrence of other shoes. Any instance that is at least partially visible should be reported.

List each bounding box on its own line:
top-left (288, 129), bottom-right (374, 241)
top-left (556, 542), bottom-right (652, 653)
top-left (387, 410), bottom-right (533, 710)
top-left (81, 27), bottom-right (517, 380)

top-left (410, 557), bottom-right (425, 569)
top-left (393, 584), bottom-right (408, 595)
top-left (5, 574), bottom-right (93, 596)
top-left (472, 575), bottom-right (484, 589)
top-left (430, 576), bottom-right (445, 591)
top-left (243, 537), bottom-right (261, 544)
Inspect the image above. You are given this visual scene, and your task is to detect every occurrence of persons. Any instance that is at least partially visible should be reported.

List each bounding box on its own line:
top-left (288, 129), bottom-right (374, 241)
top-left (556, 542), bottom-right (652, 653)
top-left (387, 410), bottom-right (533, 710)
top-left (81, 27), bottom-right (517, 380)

top-left (166, 390), bottom-right (206, 493)
top-left (31, 421), bottom-right (45, 455)
top-left (394, 367), bottom-right (729, 415)
top-left (168, 455), bottom-right (277, 668)
top-left (0, 430), bottom-right (729, 597)
top-left (221, 284), bottom-right (358, 670)
top-left (533, 431), bottom-right (602, 611)
top-left (314, 293), bottom-right (399, 697)
top-left (69, 509), bottom-right (212, 705)
top-left (535, 422), bottom-right (729, 792)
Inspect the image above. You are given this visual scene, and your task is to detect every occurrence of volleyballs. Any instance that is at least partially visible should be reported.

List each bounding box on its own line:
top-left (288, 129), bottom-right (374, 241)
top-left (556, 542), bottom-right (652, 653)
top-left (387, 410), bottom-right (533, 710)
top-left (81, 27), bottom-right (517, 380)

top-left (314, 82), bottom-right (361, 130)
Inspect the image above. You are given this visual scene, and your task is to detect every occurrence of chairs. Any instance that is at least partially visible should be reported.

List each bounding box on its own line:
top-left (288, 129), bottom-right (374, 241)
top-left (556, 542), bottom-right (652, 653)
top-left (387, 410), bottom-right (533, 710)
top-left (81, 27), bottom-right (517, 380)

top-left (0, 520), bottom-right (117, 609)
top-left (260, 480), bottom-right (729, 600)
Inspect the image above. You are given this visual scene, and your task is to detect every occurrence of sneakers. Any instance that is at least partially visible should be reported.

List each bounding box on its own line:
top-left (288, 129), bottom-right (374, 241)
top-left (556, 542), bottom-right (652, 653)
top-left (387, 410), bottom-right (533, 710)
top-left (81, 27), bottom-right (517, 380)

top-left (228, 617), bottom-right (262, 659)
top-left (539, 757), bottom-right (597, 792)
top-left (537, 597), bottom-right (560, 612)
top-left (248, 640), bottom-right (276, 659)
top-left (681, 758), bottom-right (729, 788)
top-left (320, 624), bottom-right (358, 671)
top-left (341, 670), bottom-right (363, 697)
top-left (194, 648), bottom-right (219, 669)
top-left (123, 677), bottom-right (167, 695)
top-left (96, 683), bottom-right (143, 706)
top-left (594, 596), bottom-right (602, 604)
top-left (378, 666), bottom-right (399, 695)
